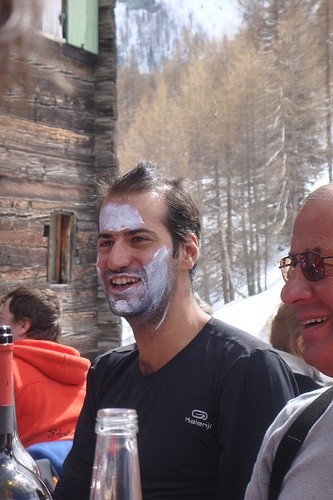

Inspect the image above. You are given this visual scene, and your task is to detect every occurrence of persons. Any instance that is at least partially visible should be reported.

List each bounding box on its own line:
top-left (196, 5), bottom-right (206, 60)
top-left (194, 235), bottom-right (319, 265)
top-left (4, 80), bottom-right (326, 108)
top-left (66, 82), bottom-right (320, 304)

top-left (51, 160), bottom-right (299, 500)
top-left (0, 285), bottom-right (91, 449)
top-left (244, 183), bottom-right (333, 500)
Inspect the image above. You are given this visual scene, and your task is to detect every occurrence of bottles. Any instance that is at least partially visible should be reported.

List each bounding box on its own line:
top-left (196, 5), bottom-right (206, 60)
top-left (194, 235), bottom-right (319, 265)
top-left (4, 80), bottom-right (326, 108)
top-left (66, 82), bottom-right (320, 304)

top-left (89, 408), bottom-right (142, 500)
top-left (0, 334), bottom-right (53, 500)
top-left (0, 325), bottom-right (41, 479)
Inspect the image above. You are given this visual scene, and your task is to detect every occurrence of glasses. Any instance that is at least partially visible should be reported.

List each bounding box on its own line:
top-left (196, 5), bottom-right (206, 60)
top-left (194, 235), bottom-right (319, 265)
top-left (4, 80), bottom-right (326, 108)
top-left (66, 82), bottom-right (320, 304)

top-left (279, 252), bottom-right (333, 283)
